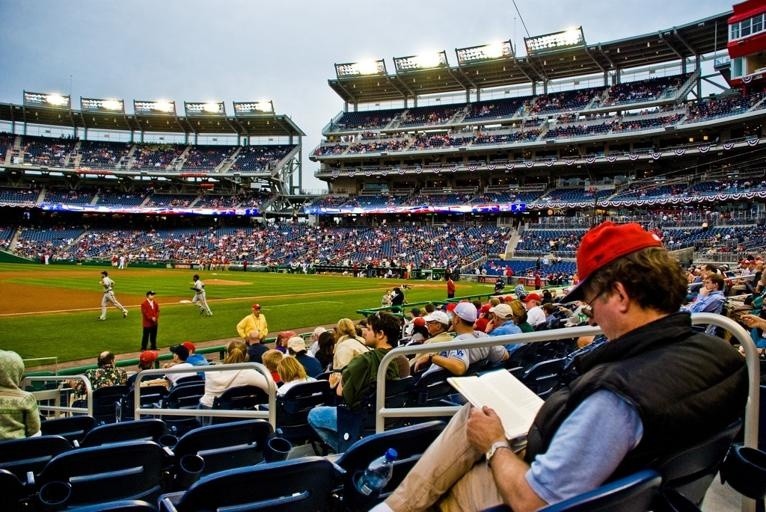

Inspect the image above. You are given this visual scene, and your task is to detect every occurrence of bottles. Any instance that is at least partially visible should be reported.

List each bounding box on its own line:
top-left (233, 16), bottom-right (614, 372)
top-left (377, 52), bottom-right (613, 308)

top-left (356, 448), bottom-right (398, 496)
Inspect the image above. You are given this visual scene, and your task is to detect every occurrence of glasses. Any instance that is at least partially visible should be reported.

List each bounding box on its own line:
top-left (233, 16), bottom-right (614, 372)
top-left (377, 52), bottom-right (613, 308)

top-left (581, 290), bottom-right (606, 318)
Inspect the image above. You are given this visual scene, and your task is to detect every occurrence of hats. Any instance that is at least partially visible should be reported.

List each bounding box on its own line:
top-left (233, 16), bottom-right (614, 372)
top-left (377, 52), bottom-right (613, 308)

top-left (559, 222), bottom-right (663, 303)
top-left (410, 296), bottom-right (525, 327)
top-left (252, 304), bottom-right (260, 309)
top-left (140, 351), bottom-right (158, 366)
top-left (288, 337), bottom-right (307, 352)
top-left (170, 342), bottom-right (195, 357)
top-left (523, 294), bottom-right (541, 302)
top-left (147, 291), bottom-right (156, 295)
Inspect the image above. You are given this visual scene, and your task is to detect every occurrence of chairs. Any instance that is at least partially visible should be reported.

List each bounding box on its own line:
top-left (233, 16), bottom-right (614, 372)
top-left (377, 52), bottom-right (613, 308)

top-left (94, 189), bottom-right (152, 208)
top-left (0, 338), bottom-right (662, 512)
top-left (487, 231), bottom-right (587, 258)
top-left (608, 183), bottom-right (688, 201)
top-left (1, 187), bottom-right (43, 203)
top-left (43, 187), bottom-right (99, 205)
top-left (191, 194), bottom-right (273, 209)
top-left (307, 198), bottom-right (347, 209)
top-left (313, 144), bottom-right (349, 155)
top-left (663, 226), bottom-right (766, 252)
top-left (0, 130), bottom-right (17, 162)
top-left (407, 136), bottom-right (475, 151)
top-left (682, 92), bottom-right (766, 125)
top-left (227, 144), bottom-right (297, 174)
top-left (18, 225), bottom-right (85, 258)
top-left (398, 103), bottom-right (467, 128)
top-left (685, 182), bottom-right (762, 197)
top-left (411, 194), bottom-right (475, 207)
top-left (143, 194), bottom-right (198, 209)
top-left (612, 113), bottom-right (685, 132)
top-left (331, 108), bottom-right (405, 132)
top-left (23, 137), bottom-right (79, 167)
top-left (180, 145), bottom-right (241, 171)
top-left (340, 197), bottom-right (408, 210)
top-left (461, 95), bottom-right (538, 123)
top-left (347, 140), bottom-right (405, 154)
top-left (2, 227), bottom-right (17, 241)
top-left (60, 224), bottom-right (514, 274)
top-left (469, 191), bottom-right (545, 205)
top-left (535, 85), bottom-right (611, 115)
top-left (130, 144), bottom-right (189, 170)
top-left (542, 124), bottom-right (613, 139)
top-left (80, 141), bottom-right (128, 169)
top-left (464, 259), bottom-right (576, 279)
top-left (601, 72), bottom-right (693, 107)
top-left (536, 188), bottom-right (617, 204)
top-left (471, 130), bottom-right (544, 145)
